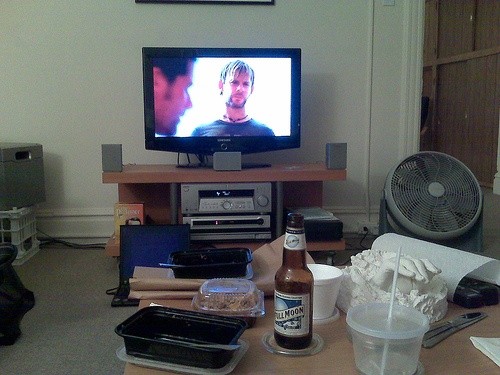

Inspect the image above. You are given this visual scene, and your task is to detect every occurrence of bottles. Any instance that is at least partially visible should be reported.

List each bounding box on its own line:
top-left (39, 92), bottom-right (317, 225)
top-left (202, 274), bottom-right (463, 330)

top-left (273, 213), bottom-right (314, 351)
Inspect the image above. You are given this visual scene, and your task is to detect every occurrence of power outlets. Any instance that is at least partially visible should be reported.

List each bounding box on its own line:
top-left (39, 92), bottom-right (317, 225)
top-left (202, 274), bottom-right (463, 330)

top-left (359, 223), bottom-right (372, 235)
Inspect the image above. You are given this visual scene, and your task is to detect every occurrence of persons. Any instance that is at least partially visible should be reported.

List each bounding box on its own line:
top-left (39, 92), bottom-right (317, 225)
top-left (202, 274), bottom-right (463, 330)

top-left (153, 55), bottom-right (197, 135)
top-left (192, 60), bottom-right (276, 136)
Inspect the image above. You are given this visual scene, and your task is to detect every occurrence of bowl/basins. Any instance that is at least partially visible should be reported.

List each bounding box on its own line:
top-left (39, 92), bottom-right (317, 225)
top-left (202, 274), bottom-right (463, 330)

top-left (307, 263), bottom-right (345, 320)
top-left (190, 277), bottom-right (266, 329)
top-left (168, 247), bottom-right (253, 279)
top-left (113, 306), bottom-right (248, 370)
top-left (345, 302), bottom-right (430, 375)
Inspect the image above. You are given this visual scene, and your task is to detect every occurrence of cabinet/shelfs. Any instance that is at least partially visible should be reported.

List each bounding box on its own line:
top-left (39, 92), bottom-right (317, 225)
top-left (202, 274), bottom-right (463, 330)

top-left (102, 162), bottom-right (347, 266)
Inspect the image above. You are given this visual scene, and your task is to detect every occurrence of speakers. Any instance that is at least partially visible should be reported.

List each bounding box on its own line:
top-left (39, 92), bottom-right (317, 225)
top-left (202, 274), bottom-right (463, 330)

top-left (102, 144), bottom-right (123, 172)
top-left (326, 143), bottom-right (348, 169)
top-left (213, 151), bottom-right (242, 171)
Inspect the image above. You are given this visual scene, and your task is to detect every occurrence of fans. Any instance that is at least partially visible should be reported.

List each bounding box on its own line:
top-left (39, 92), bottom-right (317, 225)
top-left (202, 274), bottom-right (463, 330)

top-left (377, 152), bottom-right (485, 254)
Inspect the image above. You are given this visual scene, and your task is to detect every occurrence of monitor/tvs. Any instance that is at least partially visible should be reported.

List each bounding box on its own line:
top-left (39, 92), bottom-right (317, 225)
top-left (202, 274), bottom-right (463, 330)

top-left (141, 46), bottom-right (301, 170)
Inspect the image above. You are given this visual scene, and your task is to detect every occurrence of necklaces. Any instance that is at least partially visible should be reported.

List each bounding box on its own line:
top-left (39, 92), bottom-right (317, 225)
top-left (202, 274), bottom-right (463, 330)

top-left (223, 114), bottom-right (248, 122)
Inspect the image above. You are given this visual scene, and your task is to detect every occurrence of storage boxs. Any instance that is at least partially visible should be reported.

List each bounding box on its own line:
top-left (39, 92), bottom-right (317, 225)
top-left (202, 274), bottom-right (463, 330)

top-left (0, 206), bottom-right (40, 266)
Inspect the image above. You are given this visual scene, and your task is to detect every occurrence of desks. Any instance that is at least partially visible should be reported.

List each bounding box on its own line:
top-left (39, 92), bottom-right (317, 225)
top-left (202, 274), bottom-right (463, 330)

top-left (125, 261), bottom-right (500, 375)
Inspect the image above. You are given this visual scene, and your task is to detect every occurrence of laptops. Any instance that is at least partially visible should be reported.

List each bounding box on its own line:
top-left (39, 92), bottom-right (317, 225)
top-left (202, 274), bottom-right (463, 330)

top-left (110, 225), bottom-right (191, 306)
